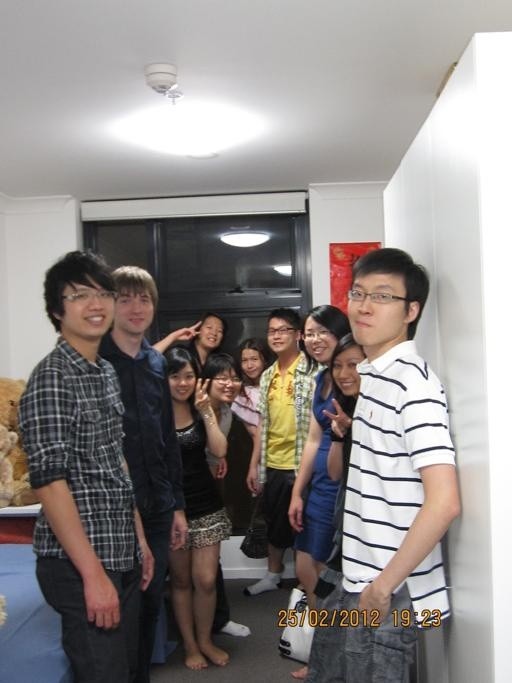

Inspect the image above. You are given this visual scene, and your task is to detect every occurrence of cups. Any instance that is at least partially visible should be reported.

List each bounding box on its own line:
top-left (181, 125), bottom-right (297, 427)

top-left (301, 330), bottom-right (332, 340)
top-left (208, 376), bottom-right (243, 386)
top-left (60, 291), bottom-right (117, 304)
top-left (347, 290), bottom-right (416, 304)
top-left (266, 325), bottom-right (297, 336)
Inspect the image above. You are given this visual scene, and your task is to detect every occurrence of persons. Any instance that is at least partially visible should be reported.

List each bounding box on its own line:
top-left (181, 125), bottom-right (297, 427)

top-left (200, 351), bottom-right (252, 637)
top-left (313, 331), bottom-right (367, 609)
top-left (303, 248), bottom-right (461, 683)
top-left (97, 266), bottom-right (191, 682)
top-left (163, 348), bottom-right (230, 670)
top-left (246, 309), bottom-right (316, 596)
top-left (152, 313), bottom-right (230, 372)
top-left (18, 247), bottom-right (157, 683)
top-left (289, 303), bottom-right (350, 679)
top-left (230, 336), bottom-right (278, 433)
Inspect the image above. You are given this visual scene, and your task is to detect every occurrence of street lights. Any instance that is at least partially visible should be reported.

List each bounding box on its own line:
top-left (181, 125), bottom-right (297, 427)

top-left (278, 586), bottom-right (316, 663)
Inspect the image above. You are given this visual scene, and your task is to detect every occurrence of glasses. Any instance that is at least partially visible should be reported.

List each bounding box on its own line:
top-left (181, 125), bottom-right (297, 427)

top-left (219, 226), bottom-right (270, 249)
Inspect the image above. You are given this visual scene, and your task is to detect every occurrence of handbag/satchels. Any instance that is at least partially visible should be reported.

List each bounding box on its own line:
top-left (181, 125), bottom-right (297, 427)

top-left (330, 431), bottom-right (346, 443)
top-left (201, 408), bottom-right (216, 426)
